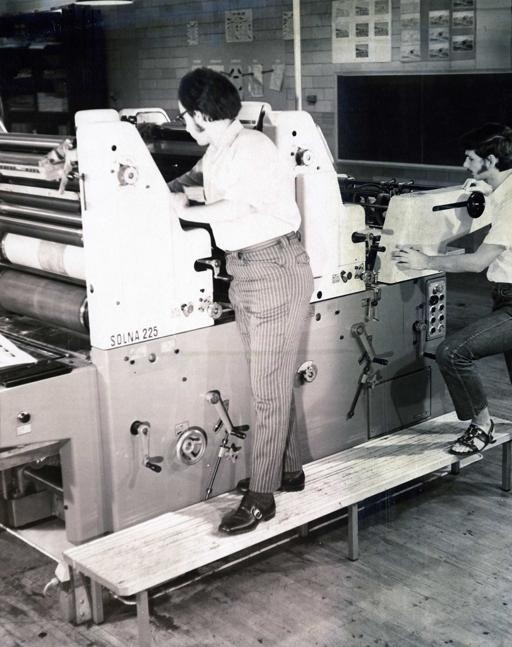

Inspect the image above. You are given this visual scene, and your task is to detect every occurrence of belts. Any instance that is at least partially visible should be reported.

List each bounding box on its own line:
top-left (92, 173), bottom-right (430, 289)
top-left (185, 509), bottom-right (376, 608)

top-left (489, 279), bottom-right (512, 295)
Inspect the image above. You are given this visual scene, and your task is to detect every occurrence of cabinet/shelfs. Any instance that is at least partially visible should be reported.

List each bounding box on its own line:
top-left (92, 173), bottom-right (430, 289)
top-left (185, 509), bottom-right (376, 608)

top-left (0, 8), bottom-right (111, 139)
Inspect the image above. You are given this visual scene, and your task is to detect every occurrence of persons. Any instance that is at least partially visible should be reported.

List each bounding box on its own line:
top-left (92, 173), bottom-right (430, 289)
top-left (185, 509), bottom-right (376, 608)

top-left (392, 123), bottom-right (512, 454)
top-left (163, 67), bottom-right (315, 536)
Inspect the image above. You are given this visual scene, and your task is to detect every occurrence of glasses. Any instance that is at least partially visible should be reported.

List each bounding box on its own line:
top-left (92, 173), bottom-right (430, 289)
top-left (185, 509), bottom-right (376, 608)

top-left (173, 106), bottom-right (194, 129)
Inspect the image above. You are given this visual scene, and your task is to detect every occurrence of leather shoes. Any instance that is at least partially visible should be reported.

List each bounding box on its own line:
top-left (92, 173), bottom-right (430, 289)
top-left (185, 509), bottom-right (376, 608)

top-left (235, 470), bottom-right (306, 496)
top-left (216, 495), bottom-right (276, 535)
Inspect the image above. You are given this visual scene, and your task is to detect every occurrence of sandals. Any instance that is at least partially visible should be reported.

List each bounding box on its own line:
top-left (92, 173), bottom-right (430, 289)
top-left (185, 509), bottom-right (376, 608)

top-left (448, 417), bottom-right (498, 454)
